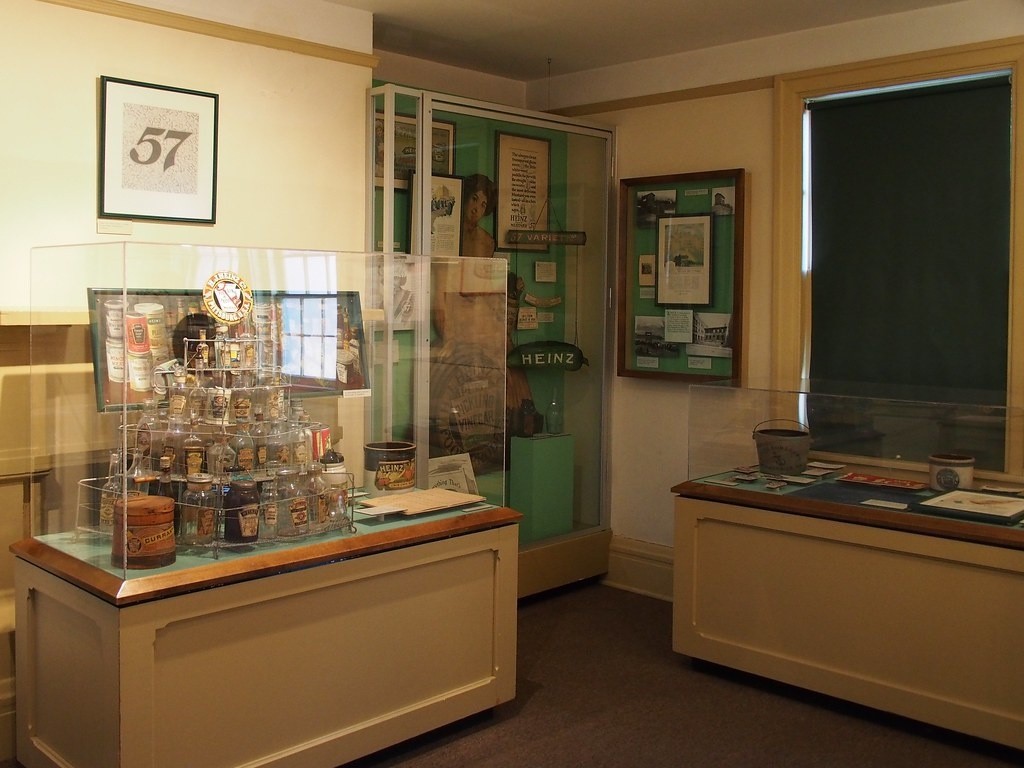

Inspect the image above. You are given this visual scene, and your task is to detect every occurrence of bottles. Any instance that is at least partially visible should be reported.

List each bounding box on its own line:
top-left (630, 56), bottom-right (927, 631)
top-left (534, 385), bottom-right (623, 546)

top-left (517, 398), bottom-right (537, 437)
top-left (545, 385), bottom-right (562, 434)
top-left (98, 328), bottom-right (347, 545)
top-left (165, 300), bottom-right (207, 360)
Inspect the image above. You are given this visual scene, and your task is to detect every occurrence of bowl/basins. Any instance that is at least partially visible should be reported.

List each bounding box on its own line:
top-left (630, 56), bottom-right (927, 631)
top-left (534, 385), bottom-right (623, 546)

top-left (930, 453), bottom-right (975, 493)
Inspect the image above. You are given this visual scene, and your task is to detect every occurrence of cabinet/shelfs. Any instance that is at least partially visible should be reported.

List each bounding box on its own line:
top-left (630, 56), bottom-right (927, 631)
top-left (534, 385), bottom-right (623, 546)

top-left (670, 458), bottom-right (1024, 752)
top-left (8, 487), bottom-right (524, 768)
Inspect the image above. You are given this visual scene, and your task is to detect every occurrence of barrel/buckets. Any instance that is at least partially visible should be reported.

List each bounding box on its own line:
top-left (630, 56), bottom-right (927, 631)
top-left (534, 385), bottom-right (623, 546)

top-left (750, 417), bottom-right (816, 476)
top-left (110, 494), bottom-right (176, 570)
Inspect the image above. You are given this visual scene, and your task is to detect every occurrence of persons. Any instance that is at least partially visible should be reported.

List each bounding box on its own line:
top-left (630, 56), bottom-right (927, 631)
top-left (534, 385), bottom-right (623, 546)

top-left (432, 175), bottom-right (533, 409)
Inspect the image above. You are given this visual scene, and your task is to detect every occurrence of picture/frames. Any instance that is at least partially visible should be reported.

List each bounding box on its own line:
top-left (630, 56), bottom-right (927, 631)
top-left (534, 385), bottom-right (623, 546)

top-left (89, 289), bottom-right (371, 414)
top-left (493, 129), bottom-right (552, 254)
top-left (654, 212), bottom-right (714, 307)
top-left (373, 251), bottom-right (415, 330)
top-left (907, 488), bottom-right (1024, 525)
top-left (375, 110), bottom-right (457, 193)
top-left (99, 74), bottom-right (220, 224)
top-left (405, 170), bottom-right (466, 257)
